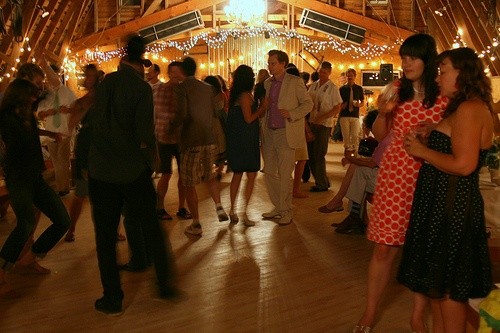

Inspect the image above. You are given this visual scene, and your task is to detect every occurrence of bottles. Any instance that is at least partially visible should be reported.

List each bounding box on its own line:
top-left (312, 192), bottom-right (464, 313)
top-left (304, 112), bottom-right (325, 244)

top-left (377, 80), bottom-right (400, 112)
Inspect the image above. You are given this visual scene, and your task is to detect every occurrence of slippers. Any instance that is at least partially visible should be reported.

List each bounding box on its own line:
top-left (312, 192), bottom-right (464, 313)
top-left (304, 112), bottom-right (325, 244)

top-left (177, 208), bottom-right (192, 219)
top-left (352, 324), bottom-right (373, 333)
top-left (155, 208), bottom-right (172, 220)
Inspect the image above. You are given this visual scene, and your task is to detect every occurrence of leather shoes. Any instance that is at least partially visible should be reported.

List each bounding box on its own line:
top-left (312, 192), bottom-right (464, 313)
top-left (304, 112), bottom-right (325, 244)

top-left (262, 210), bottom-right (281, 218)
top-left (278, 208), bottom-right (292, 224)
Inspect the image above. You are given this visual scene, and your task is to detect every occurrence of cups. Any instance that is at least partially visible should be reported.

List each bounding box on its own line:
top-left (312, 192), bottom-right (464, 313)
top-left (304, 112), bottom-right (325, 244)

top-left (353, 101), bottom-right (357, 107)
top-left (280, 108), bottom-right (288, 119)
top-left (409, 123), bottom-right (429, 145)
top-left (345, 136), bottom-right (357, 156)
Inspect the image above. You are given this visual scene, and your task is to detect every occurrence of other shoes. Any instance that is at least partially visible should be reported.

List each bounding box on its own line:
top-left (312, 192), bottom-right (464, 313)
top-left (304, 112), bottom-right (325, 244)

top-left (230, 211), bottom-right (239, 222)
top-left (116, 233), bottom-right (126, 241)
top-left (118, 261), bottom-right (146, 272)
top-left (186, 224), bottom-right (202, 235)
top-left (161, 286), bottom-right (174, 298)
top-left (243, 214), bottom-right (255, 226)
top-left (216, 204), bottom-right (229, 222)
top-left (331, 217), bottom-right (365, 235)
top-left (319, 201), bottom-right (344, 213)
top-left (0, 278), bottom-right (21, 298)
top-left (11, 261), bottom-right (50, 274)
top-left (95, 298), bottom-right (123, 317)
top-left (310, 186), bottom-right (328, 192)
top-left (65, 233), bottom-right (73, 241)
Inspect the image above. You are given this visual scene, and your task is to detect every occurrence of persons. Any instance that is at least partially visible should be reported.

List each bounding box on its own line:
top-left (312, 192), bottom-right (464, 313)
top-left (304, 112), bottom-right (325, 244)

top-left (350, 34), bottom-right (451, 333)
top-left (0, 36), bottom-right (382, 314)
top-left (398, 46), bottom-right (500, 333)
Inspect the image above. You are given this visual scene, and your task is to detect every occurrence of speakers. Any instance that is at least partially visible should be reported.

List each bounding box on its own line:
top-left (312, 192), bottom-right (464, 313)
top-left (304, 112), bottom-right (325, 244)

top-left (380, 64), bottom-right (393, 83)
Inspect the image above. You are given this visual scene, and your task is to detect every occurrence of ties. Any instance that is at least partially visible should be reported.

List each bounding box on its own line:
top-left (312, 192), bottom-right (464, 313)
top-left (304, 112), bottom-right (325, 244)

top-left (349, 87), bottom-right (353, 112)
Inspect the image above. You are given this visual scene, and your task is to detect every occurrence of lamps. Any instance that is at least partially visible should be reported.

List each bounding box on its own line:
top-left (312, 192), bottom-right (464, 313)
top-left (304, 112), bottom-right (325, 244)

top-left (36, 3), bottom-right (49, 19)
